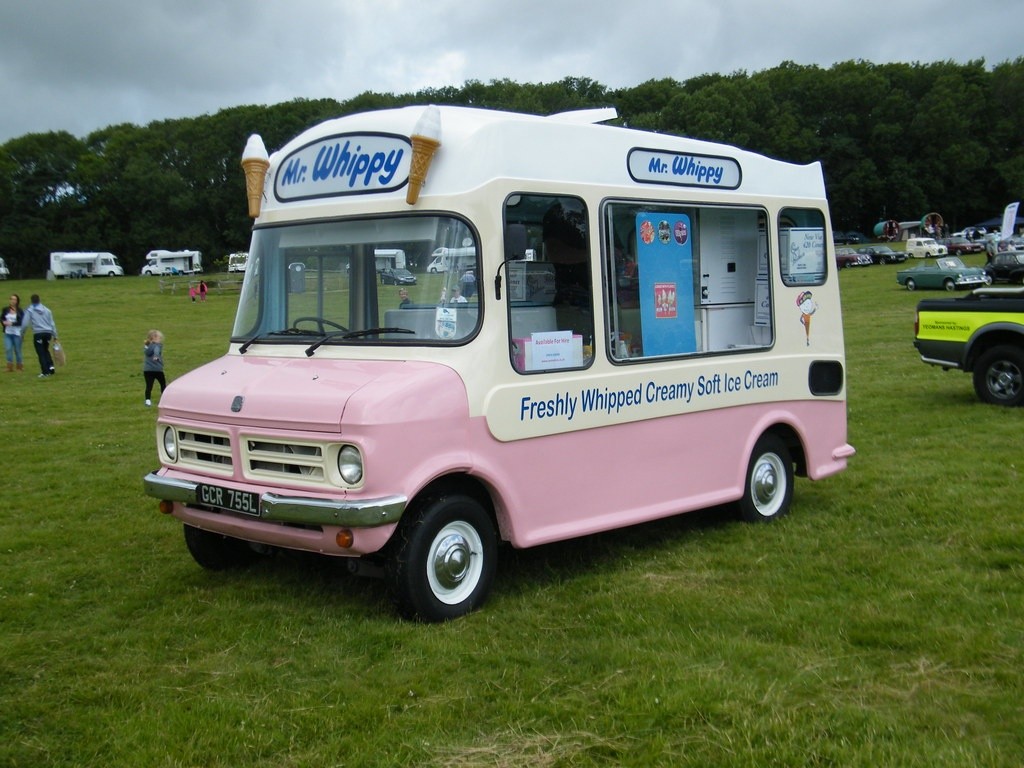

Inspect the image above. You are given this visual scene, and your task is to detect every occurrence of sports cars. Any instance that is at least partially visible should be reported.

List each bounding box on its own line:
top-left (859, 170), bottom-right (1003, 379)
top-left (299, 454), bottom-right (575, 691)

top-left (896, 256), bottom-right (993, 293)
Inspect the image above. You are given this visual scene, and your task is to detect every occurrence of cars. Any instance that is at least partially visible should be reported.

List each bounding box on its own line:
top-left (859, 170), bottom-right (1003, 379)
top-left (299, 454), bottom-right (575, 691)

top-left (981, 232), bottom-right (1024, 285)
top-left (835, 247), bottom-right (874, 270)
top-left (906, 237), bottom-right (948, 259)
top-left (914, 287), bottom-right (1024, 408)
top-left (857, 246), bottom-right (908, 266)
top-left (937, 237), bottom-right (986, 257)
top-left (380, 267), bottom-right (417, 286)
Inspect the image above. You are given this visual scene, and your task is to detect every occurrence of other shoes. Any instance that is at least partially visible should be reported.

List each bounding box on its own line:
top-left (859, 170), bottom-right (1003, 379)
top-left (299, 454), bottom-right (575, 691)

top-left (49, 365), bottom-right (55, 373)
top-left (39, 373), bottom-right (50, 377)
top-left (145, 399), bottom-right (151, 406)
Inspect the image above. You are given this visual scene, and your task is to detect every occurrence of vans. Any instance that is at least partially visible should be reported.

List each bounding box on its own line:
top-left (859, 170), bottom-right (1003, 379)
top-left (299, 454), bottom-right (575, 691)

top-left (426, 247), bottom-right (476, 274)
top-left (144, 109), bottom-right (854, 626)
top-left (141, 249), bottom-right (204, 277)
top-left (49, 251), bottom-right (124, 277)
top-left (228, 252), bottom-right (259, 273)
top-left (346, 249), bottom-right (406, 275)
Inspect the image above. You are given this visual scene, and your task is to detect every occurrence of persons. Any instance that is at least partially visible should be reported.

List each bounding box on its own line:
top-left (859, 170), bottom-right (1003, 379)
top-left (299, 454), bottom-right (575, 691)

top-left (399, 288), bottom-right (414, 309)
top-left (0, 294), bottom-right (25, 372)
top-left (197, 280), bottom-right (207, 302)
top-left (986, 240), bottom-right (994, 262)
top-left (143, 330), bottom-right (166, 406)
top-left (1008, 240), bottom-right (1016, 251)
top-left (189, 287), bottom-right (197, 303)
top-left (460, 271), bottom-right (475, 297)
top-left (22, 295), bottom-right (58, 377)
top-left (450, 285), bottom-right (467, 303)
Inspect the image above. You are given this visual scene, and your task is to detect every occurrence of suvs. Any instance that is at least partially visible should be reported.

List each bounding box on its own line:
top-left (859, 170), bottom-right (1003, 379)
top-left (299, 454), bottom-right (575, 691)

top-left (951, 226), bottom-right (988, 239)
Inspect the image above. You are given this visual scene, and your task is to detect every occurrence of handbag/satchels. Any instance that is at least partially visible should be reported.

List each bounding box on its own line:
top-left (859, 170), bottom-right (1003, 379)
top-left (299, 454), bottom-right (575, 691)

top-left (53, 341), bottom-right (65, 364)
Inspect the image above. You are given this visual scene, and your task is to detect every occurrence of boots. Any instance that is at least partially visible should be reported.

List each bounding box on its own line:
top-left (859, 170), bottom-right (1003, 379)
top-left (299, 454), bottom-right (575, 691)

top-left (16, 363), bottom-right (22, 373)
top-left (5, 363), bottom-right (13, 372)
